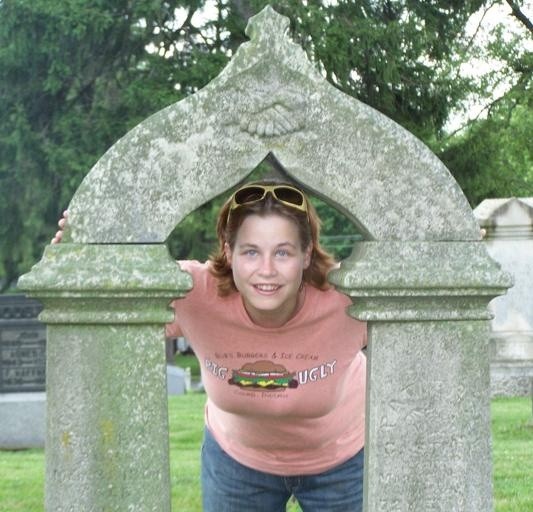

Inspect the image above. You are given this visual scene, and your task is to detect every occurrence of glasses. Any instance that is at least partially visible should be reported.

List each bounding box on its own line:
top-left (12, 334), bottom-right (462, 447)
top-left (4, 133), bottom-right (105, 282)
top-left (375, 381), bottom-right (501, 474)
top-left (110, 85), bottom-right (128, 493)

top-left (226, 185), bottom-right (309, 223)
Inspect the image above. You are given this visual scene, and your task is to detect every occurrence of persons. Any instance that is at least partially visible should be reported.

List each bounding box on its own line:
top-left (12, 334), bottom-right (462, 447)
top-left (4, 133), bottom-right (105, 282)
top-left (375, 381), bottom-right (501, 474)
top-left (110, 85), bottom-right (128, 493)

top-left (51, 180), bottom-right (487, 512)
